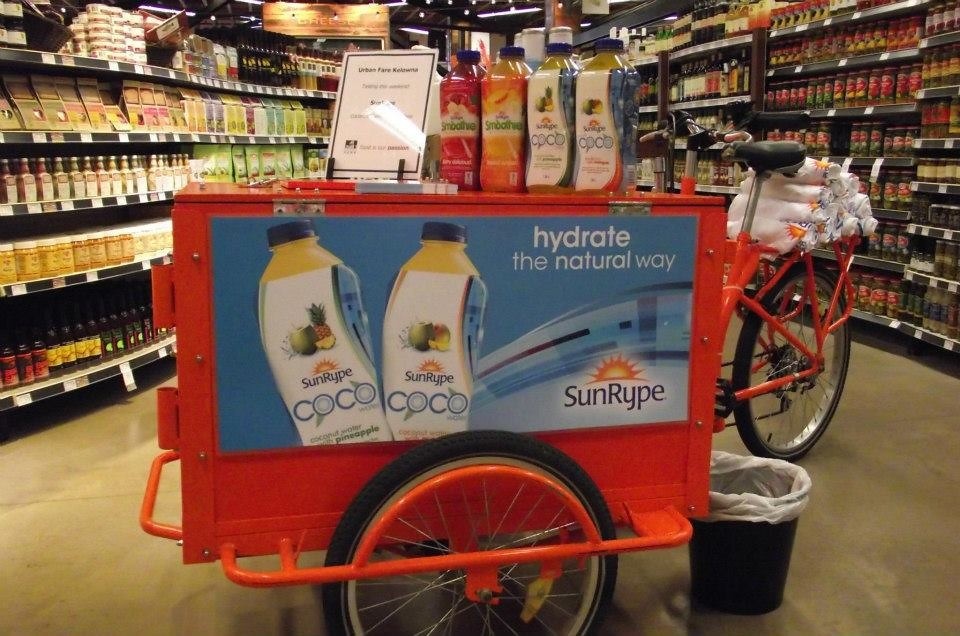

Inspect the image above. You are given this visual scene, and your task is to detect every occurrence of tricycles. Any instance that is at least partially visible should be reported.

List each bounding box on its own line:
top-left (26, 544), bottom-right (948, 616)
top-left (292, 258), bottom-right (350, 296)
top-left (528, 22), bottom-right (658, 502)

top-left (139, 99), bottom-right (873, 636)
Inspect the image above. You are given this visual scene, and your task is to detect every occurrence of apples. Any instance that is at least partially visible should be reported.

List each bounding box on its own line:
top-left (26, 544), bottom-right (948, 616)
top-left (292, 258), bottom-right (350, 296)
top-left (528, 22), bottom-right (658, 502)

top-left (582, 99), bottom-right (603, 114)
top-left (536, 96), bottom-right (545, 112)
top-left (407, 320), bottom-right (450, 351)
top-left (290, 325), bottom-right (319, 354)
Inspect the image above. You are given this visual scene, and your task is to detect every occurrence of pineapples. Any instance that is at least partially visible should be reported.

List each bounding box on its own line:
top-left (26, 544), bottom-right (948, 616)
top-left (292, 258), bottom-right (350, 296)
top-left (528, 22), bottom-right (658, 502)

top-left (544, 86), bottom-right (554, 106)
top-left (305, 303), bottom-right (336, 349)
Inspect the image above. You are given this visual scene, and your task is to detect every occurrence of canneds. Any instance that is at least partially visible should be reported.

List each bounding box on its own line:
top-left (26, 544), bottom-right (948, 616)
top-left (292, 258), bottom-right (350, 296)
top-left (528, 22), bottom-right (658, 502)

top-left (765, 64), bottom-right (916, 319)
top-left (910, 1), bottom-right (960, 282)
top-left (0, 227), bottom-right (135, 287)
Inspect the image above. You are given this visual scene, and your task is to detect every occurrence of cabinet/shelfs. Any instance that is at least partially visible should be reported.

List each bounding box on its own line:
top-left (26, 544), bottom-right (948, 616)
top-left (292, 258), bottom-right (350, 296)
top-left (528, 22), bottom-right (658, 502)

top-left (0, 0), bottom-right (960, 411)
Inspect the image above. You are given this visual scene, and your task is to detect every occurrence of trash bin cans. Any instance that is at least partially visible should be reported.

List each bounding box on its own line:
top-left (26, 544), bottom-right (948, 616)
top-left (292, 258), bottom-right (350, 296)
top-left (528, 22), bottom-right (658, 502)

top-left (690, 457), bottom-right (811, 615)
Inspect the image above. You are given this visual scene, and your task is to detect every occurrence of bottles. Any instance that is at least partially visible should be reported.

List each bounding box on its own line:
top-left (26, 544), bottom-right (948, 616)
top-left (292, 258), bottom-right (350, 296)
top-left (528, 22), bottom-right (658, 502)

top-left (439, 50), bottom-right (488, 191)
top-left (258, 219), bottom-right (392, 446)
top-left (0, 280), bottom-right (154, 386)
top-left (574, 36), bottom-right (640, 197)
top-left (237, 39), bottom-right (343, 92)
top-left (0, 229), bottom-right (136, 285)
top-left (909, 238), bottom-right (960, 282)
top-left (895, 278), bottom-right (960, 342)
top-left (381, 221), bottom-right (487, 442)
top-left (0, 153), bottom-right (191, 204)
top-left (409, 45), bottom-right (443, 180)
top-left (480, 46), bottom-right (534, 193)
top-left (609, 0), bottom-right (960, 186)
top-left (526, 42), bottom-right (580, 195)
